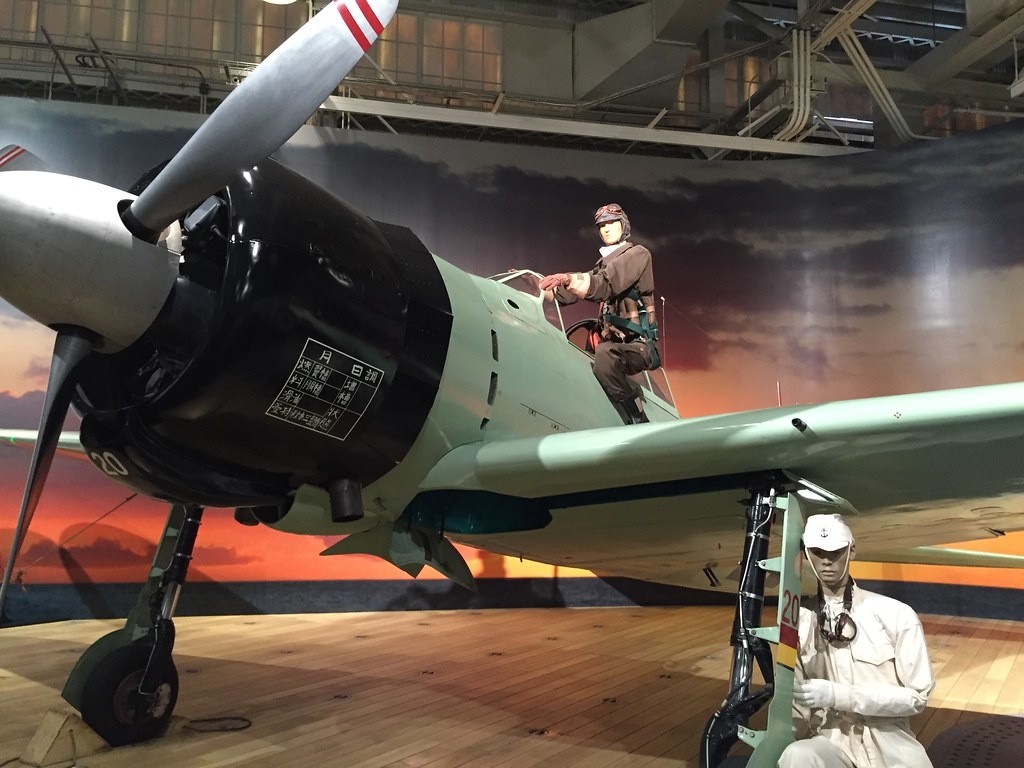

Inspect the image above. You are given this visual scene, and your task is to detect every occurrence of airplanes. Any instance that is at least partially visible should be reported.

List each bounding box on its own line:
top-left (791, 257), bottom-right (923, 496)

top-left (0, 0), bottom-right (1024, 768)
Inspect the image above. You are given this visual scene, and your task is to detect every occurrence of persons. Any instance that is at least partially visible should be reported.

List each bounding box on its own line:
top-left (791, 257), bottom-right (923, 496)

top-left (775, 514), bottom-right (936, 767)
top-left (507, 203), bottom-right (661, 425)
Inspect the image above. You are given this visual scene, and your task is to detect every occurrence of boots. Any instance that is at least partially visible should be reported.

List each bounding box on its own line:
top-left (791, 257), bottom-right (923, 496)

top-left (618, 391), bottom-right (648, 424)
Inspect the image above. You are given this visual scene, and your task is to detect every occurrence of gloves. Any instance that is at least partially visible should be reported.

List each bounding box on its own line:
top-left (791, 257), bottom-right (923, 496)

top-left (541, 273), bottom-right (570, 292)
top-left (793, 678), bottom-right (835, 709)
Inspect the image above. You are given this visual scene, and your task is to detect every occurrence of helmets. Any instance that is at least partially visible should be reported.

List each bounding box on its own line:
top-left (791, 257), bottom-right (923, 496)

top-left (803, 512), bottom-right (854, 552)
top-left (595, 204), bottom-right (631, 245)
